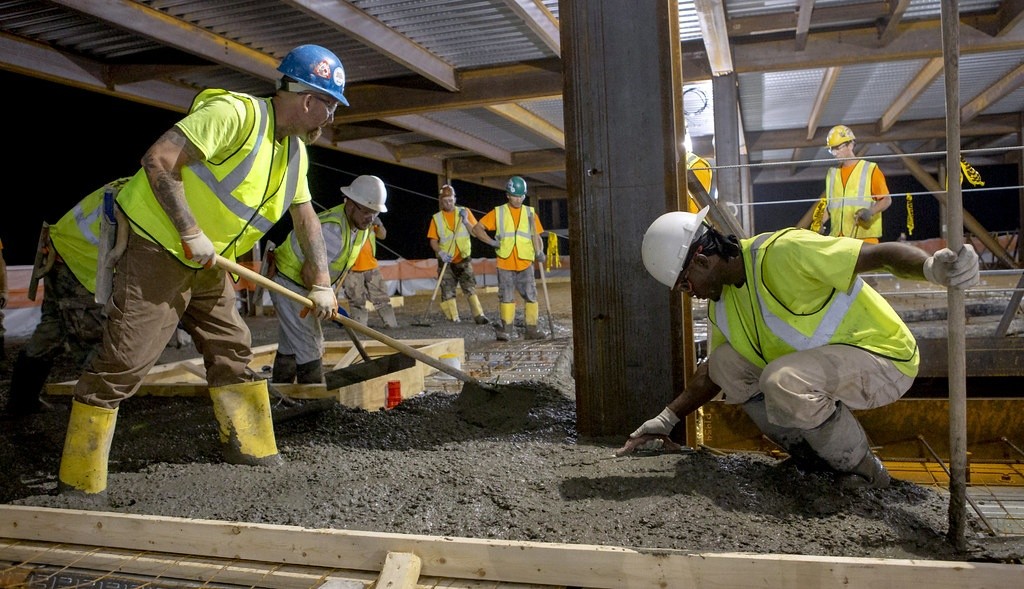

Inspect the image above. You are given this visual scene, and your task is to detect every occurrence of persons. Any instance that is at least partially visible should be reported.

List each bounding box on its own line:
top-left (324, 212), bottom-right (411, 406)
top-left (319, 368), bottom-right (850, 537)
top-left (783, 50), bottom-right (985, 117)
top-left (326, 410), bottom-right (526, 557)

top-left (810, 126), bottom-right (891, 244)
top-left (631, 204), bottom-right (980, 489)
top-left (428, 176), bottom-right (545, 337)
top-left (0, 45), bottom-right (398, 498)
top-left (686, 154), bottom-right (713, 212)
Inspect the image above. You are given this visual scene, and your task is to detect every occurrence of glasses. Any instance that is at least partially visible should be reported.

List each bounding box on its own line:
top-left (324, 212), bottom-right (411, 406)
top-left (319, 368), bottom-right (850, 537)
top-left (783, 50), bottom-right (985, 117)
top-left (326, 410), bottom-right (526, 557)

top-left (828, 142), bottom-right (851, 155)
top-left (296, 90), bottom-right (338, 113)
top-left (351, 199), bottom-right (380, 217)
top-left (679, 243), bottom-right (705, 294)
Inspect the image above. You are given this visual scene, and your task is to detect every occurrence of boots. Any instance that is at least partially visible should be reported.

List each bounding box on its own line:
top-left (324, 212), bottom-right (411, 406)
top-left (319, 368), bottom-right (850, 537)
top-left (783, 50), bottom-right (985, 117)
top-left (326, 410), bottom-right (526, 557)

top-left (524, 301), bottom-right (547, 340)
top-left (742, 394), bottom-right (830, 472)
top-left (296, 357), bottom-right (323, 384)
top-left (58, 396), bottom-right (119, 494)
top-left (468, 294), bottom-right (488, 324)
top-left (272, 349), bottom-right (299, 385)
top-left (8, 348), bottom-right (54, 415)
top-left (500, 301), bottom-right (515, 340)
top-left (440, 297), bottom-right (460, 322)
top-left (799, 399), bottom-right (892, 515)
top-left (208, 379), bottom-right (282, 467)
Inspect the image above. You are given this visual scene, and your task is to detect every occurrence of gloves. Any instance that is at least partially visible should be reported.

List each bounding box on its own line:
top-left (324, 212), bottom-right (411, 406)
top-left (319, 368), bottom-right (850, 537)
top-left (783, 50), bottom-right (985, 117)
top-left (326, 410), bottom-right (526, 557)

top-left (439, 249), bottom-right (453, 264)
top-left (180, 232), bottom-right (217, 270)
top-left (299, 285), bottom-right (339, 321)
top-left (458, 208), bottom-right (468, 220)
top-left (490, 239), bottom-right (502, 248)
top-left (923, 246), bottom-right (979, 291)
top-left (857, 208), bottom-right (874, 225)
top-left (630, 404), bottom-right (679, 450)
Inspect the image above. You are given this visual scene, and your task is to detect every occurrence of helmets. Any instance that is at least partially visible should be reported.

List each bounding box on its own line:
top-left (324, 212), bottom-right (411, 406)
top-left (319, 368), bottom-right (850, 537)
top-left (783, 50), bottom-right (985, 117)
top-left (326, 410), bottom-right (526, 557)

top-left (275, 43), bottom-right (351, 106)
top-left (641, 204), bottom-right (711, 290)
top-left (440, 184), bottom-right (456, 197)
top-left (506, 175), bottom-right (528, 197)
top-left (341, 174), bottom-right (388, 213)
top-left (824, 125), bottom-right (857, 149)
